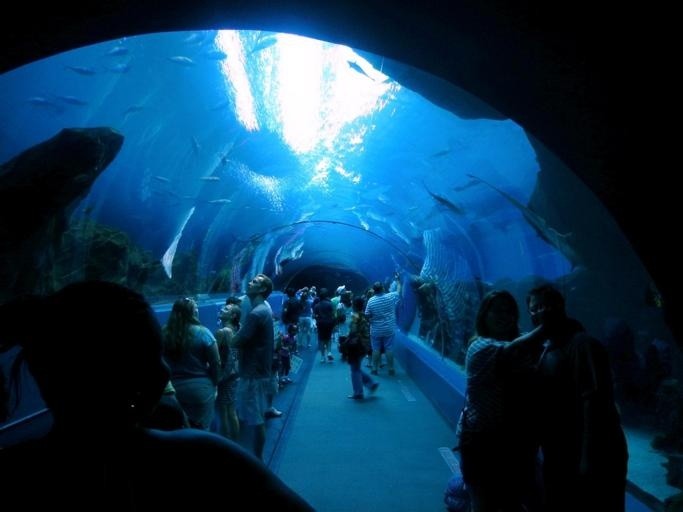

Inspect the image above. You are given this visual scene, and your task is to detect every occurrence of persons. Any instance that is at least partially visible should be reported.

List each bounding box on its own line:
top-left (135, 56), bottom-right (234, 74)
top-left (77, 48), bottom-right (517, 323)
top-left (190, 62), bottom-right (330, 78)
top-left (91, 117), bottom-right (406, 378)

top-left (411, 227), bottom-right (455, 270)
top-left (452, 289), bottom-right (583, 509)
top-left (162, 275), bottom-right (403, 462)
top-left (526, 282), bottom-right (626, 510)
top-left (2, 282), bottom-right (314, 510)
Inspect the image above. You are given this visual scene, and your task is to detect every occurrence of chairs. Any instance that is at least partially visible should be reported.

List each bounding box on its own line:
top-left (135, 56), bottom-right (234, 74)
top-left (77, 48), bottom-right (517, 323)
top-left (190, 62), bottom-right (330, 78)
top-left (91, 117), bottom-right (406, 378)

top-left (266, 407), bottom-right (282, 417)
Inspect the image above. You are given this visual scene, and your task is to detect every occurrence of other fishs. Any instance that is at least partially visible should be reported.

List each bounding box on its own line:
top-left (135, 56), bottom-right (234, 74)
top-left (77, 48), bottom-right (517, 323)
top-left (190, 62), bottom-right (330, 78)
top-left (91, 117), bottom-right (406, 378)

top-left (246, 34), bottom-right (276, 58)
top-left (166, 33), bottom-right (226, 70)
top-left (62, 46), bottom-right (136, 77)
top-left (151, 134), bottom-right (234, 203)
top-left (121, 103), bottom-right (144, 119)
top-left (27, 94), bottom-right (87, 113)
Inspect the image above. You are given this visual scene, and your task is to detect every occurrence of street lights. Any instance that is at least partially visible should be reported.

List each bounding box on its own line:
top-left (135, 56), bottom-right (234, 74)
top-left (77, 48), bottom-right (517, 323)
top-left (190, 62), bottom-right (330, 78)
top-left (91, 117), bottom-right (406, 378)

top-left (218, 344), bottom-right (229, 369)
